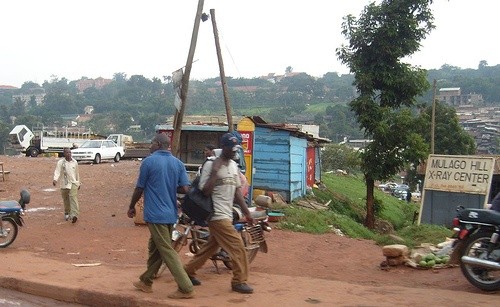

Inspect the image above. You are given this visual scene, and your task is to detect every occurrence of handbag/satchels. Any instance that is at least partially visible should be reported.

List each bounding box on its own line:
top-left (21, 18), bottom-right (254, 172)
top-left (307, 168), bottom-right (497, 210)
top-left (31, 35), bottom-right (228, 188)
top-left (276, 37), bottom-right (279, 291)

top-left (182, 176), bottom-right (215, 228)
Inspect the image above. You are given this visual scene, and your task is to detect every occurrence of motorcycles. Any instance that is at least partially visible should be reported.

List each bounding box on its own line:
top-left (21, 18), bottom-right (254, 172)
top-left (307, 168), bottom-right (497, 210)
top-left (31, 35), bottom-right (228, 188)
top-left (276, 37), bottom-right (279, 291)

top-left (0, 190), bottom-right (30, 248)
top-left (448, 205), bottom-right (499, 292)
top-left (153, 196), bottom-right (271, 279)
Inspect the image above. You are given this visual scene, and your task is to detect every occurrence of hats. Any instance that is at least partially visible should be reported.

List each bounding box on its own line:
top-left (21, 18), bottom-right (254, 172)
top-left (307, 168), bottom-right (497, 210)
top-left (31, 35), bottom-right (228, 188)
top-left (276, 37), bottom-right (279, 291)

top-left (220, 130), bottom-right (243, 152)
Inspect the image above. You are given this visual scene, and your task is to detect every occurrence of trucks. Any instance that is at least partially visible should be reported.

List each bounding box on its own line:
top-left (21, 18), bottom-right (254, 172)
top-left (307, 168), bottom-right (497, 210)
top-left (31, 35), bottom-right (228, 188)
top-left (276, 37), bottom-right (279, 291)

top-left (106, 134), bottom-right (151, 158)
top-left (8, 124), bottom-right (90, 157)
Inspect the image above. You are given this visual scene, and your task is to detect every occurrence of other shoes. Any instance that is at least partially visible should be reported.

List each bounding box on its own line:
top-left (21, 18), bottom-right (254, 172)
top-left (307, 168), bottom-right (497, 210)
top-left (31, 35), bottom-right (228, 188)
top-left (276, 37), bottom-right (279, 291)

top-left (166, 289), bottom-right (194, 298)
top-left (133, 279), bottom-right (152, 293)
top-left (188, 275), bottom-right (200, 285)
top-left (65, 214), bottom-right (70, 220)
top-left (71, 216), bottom-right (77, 223)
top-left (231, 281), bottom-right (254, 293)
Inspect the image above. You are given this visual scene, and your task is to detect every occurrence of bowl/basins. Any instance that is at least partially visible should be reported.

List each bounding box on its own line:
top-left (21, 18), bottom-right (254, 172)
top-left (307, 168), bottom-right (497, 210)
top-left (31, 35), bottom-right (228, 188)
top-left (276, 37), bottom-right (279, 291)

top-left (268, 213), bottom-right (285, 221)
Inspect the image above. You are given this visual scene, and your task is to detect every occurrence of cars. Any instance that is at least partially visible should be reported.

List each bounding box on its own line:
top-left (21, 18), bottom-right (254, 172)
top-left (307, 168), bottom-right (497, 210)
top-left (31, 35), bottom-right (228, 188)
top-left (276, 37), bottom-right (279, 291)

top-left (378, 183), bottom-right (398, 190)
top-left (71, 140), bottom-right (125, 164)
top-left (390, 184), bottom-right (410, 192)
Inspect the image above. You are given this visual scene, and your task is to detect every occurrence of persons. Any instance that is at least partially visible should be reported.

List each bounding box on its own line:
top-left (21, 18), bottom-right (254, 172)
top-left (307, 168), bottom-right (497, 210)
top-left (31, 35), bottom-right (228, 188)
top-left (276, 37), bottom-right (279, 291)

top-left (183, 133), bottom-right (254, 293)
top-left (52, 147), bottom-right (82, 223)
top-left (126, 133), bottom-right (196, 300)
top-left (204, 144), bottom-right (215, 157)
top-left (206, 131), bottom-right (250, 230)
